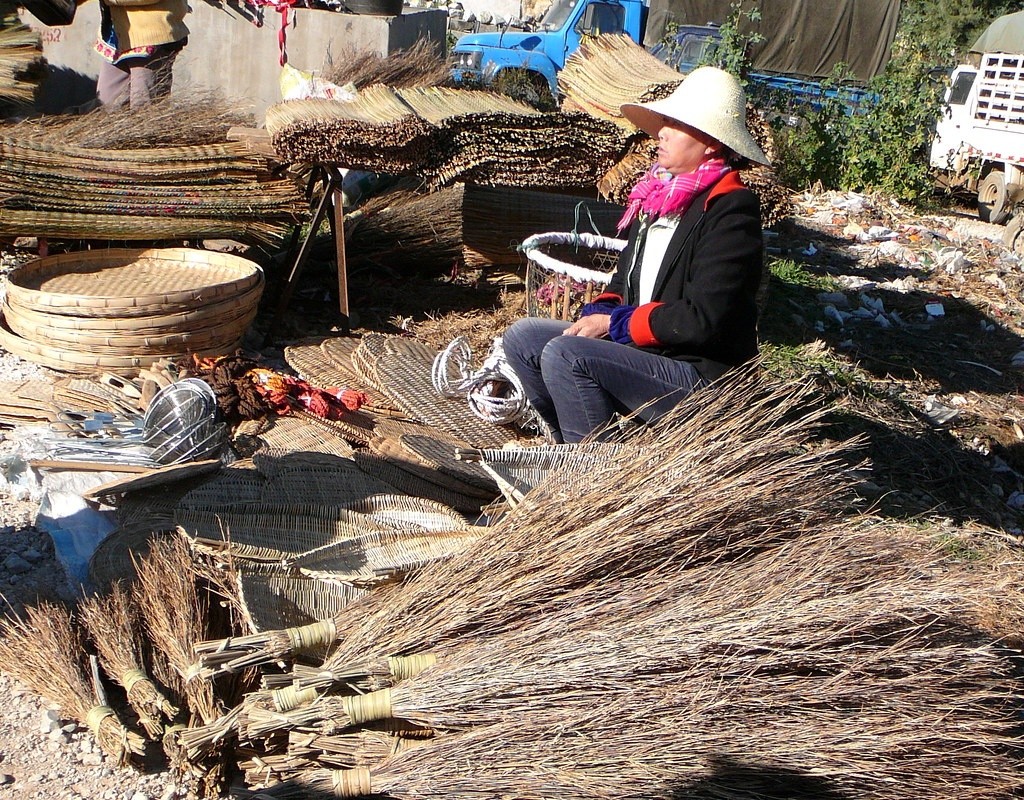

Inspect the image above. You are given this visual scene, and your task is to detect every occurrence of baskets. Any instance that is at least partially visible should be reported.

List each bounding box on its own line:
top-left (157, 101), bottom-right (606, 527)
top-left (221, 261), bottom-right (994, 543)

top-left (463, 181), bottom-right (631, 267)
top-left (1, 249), bottom-right (265, 378)
top-left (523, 231), bottom-right (628, 326)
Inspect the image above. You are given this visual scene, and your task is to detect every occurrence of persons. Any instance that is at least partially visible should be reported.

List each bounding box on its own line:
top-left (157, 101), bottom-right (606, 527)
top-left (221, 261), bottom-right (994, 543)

top-left (503, 65), bottom-right (773, 449)
top-left (93, 1), bottom-right (189, 116)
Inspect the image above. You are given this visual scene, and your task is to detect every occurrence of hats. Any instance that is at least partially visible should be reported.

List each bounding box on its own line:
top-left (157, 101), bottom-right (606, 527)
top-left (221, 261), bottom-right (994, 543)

top-left (620, 65), bottom-right (773, 171)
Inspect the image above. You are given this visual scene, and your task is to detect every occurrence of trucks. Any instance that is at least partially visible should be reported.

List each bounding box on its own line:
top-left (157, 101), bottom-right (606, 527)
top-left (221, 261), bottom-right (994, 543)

top-left (922, 7), bottom-right (1024, 228)
top-left (649, 18), bottom-right (740, 77)
top-left (452, 0), bottom-right (892, 154)
